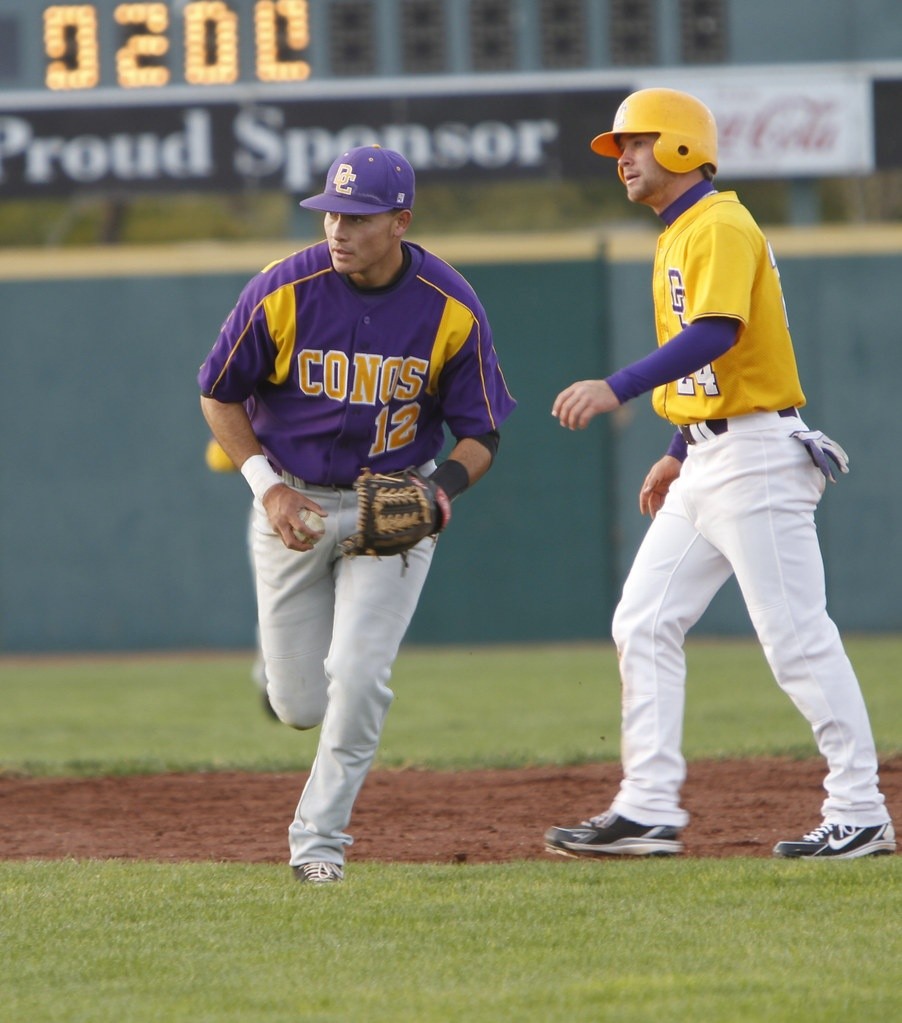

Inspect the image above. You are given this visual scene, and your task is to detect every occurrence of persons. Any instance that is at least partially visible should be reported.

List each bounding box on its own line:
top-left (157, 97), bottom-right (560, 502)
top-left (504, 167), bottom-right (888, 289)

top-left (197, 145), bottom-right (518, 886)
top-left (540, 86), bottom-right (893, 862)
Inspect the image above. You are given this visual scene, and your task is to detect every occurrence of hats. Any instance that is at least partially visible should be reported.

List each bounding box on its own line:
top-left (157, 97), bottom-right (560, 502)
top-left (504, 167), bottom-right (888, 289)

top-left (299, 143), bottom-right (414, 214)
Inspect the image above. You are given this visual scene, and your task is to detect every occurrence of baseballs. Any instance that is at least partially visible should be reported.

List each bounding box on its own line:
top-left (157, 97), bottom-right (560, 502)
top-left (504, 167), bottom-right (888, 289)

top-left (291, 508), bottom-right (325, 545)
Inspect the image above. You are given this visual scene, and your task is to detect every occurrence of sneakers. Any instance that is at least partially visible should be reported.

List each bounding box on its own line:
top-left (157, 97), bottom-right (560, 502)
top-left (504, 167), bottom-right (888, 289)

top-left (773, 818), bottom-right (897, 860)
top-left (543, 809), bottom-right (686, 858)
top-left (292, 862), bottom-right (341, 887)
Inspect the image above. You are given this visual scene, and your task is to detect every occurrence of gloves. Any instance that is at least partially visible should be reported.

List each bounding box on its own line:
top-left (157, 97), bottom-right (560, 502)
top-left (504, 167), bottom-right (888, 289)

top-left (796, 429), bottom-right (851, 482)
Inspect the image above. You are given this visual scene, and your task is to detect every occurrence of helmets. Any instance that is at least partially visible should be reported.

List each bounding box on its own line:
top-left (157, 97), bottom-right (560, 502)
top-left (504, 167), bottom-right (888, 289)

top-left (590, 85), bottom-right (718, 186)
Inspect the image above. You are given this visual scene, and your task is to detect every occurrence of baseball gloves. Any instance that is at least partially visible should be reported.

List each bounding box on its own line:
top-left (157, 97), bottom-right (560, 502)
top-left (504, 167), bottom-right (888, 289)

top-left (339, 472), bottom-right (451, 577)
